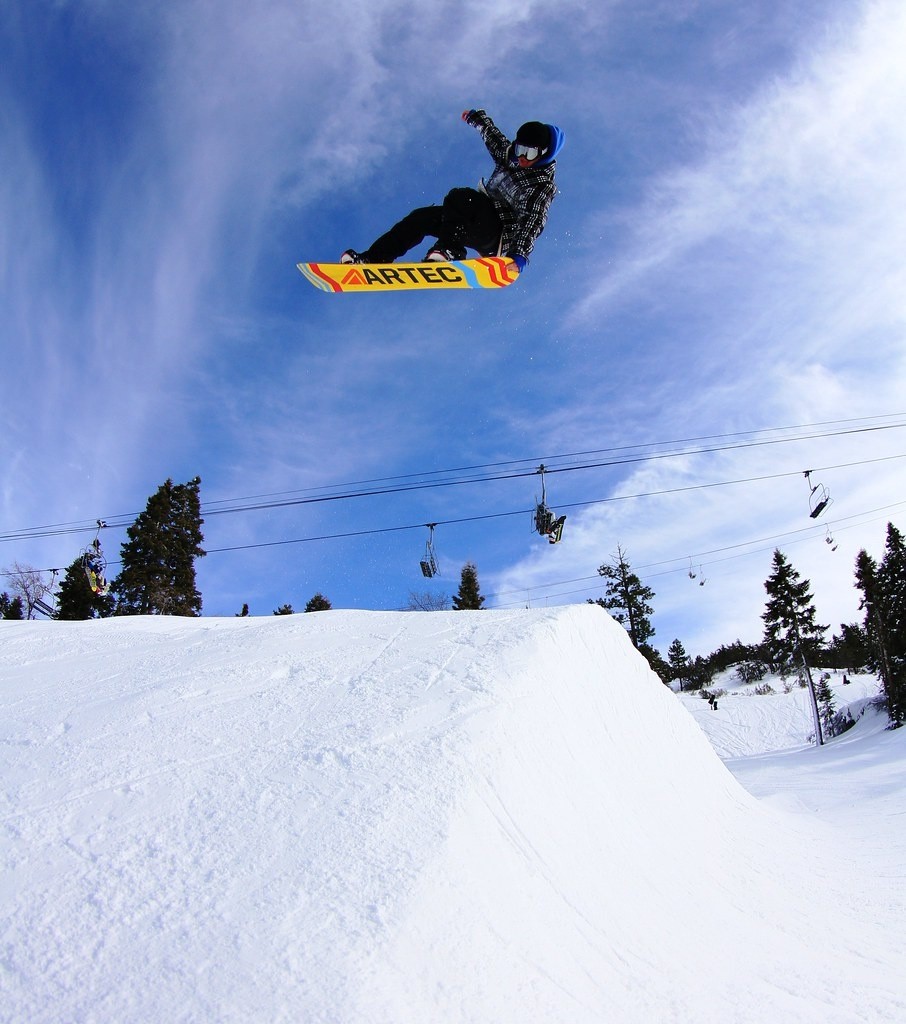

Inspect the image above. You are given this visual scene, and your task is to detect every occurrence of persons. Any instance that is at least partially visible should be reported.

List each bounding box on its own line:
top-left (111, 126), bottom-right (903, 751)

top-left (535, 500), bottom-right (566, 544)
top-left (340, 107), bottom-right (566, 275)
top-left (80, 539), bottom-right (111, 596)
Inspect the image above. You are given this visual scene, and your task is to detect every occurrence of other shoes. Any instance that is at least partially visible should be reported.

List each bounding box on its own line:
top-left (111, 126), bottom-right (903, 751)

top-left (427, 243), bottom-right (466, 261)
top-left (340, 249), bottom-right (365, 264)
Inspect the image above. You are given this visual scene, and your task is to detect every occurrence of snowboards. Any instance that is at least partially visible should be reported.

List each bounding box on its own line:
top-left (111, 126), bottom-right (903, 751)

top-left (296, 256), bottom-right (519, 293)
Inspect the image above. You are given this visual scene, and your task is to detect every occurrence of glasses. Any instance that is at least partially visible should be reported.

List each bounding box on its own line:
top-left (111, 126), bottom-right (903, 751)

top-left (515, 146), bottom-right (548, 160)
